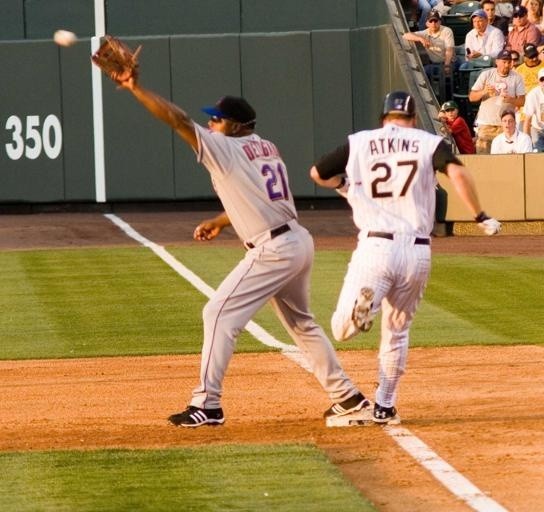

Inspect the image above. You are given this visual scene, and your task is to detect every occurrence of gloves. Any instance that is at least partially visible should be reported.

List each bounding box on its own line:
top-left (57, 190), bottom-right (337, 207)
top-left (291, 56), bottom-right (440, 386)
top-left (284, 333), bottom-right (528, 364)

top-left (475, 210), bottom-right (502, 236)
top-left (335, 177), bottom-right (349, 199)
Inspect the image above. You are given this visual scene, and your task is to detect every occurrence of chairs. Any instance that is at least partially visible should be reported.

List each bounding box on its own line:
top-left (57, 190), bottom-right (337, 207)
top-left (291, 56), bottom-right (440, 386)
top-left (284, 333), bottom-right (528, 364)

top-left (422, 0), bottom-right (493, 122)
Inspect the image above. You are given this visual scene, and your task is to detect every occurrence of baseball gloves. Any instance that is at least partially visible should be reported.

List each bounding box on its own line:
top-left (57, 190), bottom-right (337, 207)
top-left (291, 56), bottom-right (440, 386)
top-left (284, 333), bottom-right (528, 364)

top-left (92, 36), bottom-right (142, 85)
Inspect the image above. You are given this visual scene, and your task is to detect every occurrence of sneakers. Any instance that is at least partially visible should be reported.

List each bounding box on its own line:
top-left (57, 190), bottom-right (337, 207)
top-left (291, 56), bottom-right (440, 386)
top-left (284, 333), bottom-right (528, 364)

top-left (323, 391), bottom-right (371, 419)
top-left (372, 401), bottom-right (401, 422)
top-left (352, 287), bottom-right (374, 332)
top-left (167, 405), bottom-right (225, 427)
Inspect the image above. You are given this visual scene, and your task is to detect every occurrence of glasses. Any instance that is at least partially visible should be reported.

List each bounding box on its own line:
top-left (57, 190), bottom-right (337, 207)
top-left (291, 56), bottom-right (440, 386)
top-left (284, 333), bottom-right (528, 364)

top-left (513, 58), bottom-right (519, 62)
top-left (513, 14), bottom-right (525, 18)
top-left (210, 114), bottom-right (222, 123)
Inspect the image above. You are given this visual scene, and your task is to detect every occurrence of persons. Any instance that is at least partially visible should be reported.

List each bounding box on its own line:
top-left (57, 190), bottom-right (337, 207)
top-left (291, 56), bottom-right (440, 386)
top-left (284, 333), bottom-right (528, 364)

top-left (310, 91), bottom-right (502, 423)
top-left (91, 35), bottom-right (370, 428)
top-left (402, 0), bottom-right (544, 154)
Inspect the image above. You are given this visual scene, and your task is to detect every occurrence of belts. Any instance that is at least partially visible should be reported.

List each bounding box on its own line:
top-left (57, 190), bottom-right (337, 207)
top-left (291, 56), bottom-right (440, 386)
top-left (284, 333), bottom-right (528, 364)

top-left (367, 230), bottom-right (431, 245)
top-left (243, 223), bottom-right (293, 252)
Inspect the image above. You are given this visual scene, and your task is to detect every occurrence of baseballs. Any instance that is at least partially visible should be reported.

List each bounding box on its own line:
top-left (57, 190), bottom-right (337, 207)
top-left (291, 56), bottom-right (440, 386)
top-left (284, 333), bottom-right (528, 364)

top-left (54, 30), bottom-right (76, 47)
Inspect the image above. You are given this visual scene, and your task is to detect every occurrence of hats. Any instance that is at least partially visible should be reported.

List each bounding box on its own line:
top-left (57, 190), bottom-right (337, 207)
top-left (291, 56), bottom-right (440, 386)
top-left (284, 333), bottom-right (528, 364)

top-left (513, 6), bottom-right (528, 14)
top-left (524, 43), bottom-right (539, 57)
top-left (497, 50), bottom-right (512, 60)
top-left (538, 69), bottom-right (544, 82)
top-left (444, 101), bottom-right (458, 111)
top-left (471, 9), bottom-right (487, 17)
top-left (200, 95), bottom-right (256, 129)
top-left (428, 11), bottom-right (439, 21)
top-left (381, 91), bottom-right (416, 116)
